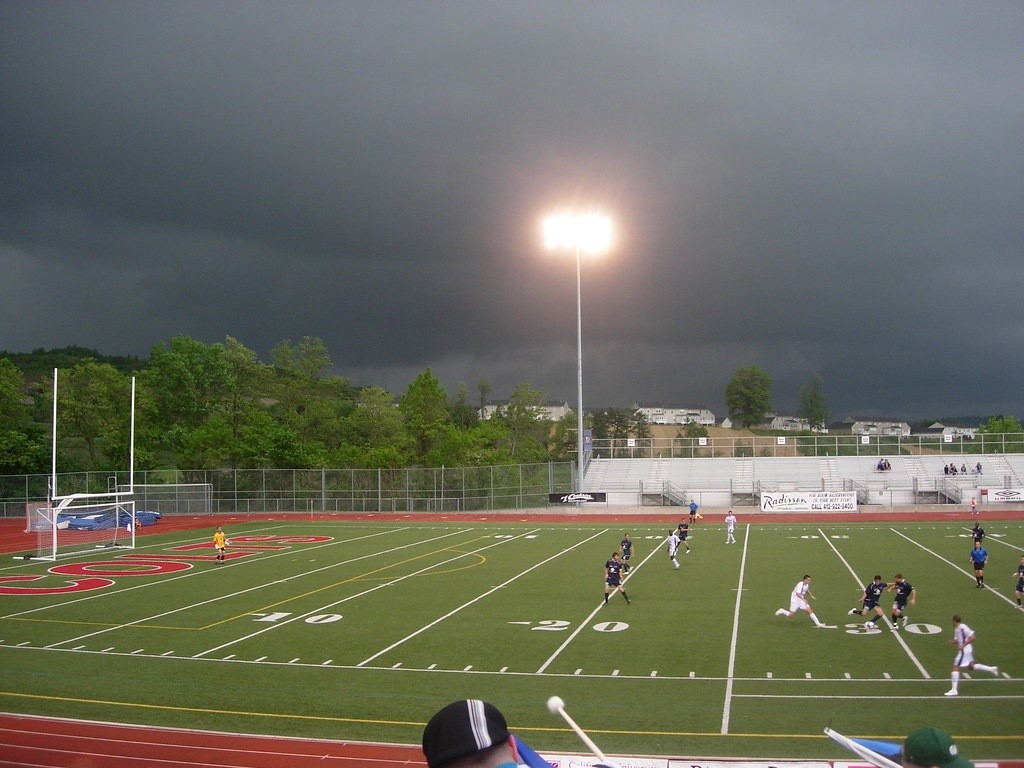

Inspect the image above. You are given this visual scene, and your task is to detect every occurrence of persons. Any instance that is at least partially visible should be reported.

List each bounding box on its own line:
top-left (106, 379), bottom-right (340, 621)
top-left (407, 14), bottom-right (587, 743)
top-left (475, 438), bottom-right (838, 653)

top-left (214, 526), bottom-right (229, 564)
top-left (1011, 555), bottom-right (1024, 608)
top-left (135, 518), bottom-right (141, 531)
top-left (877, 458), bottom-right (891, 471)
top-left (725, 511), bottom-right (737, 545)
top-left (969, 541), bottom-right (988, 589)
top-left (689, 500), bottom-right (698, 523)
top-left (677, 517), bottom-right (690, 554)
top-left (970, 497), bottom-right (979, 516)
top-left (422, 699), bottom-right (520, 768)
top-left (943, 461), bottom-right (983, 476)
top-left (604, 552), bottom-right (630, 606)
top-left (620, 533), bottom-right (634, 574)
top-left (901, 727), bottom-right (975, 768)
top-left (848, 573), bottom-right (915, 630)
top-left (775, 575), bottom-right (825, 629)
top-left (944, 615), bottom-right (998, 697)
top-left (667, 529), bottom-right (681, 570)
top-left (971, 522), bottom-right (986, 547)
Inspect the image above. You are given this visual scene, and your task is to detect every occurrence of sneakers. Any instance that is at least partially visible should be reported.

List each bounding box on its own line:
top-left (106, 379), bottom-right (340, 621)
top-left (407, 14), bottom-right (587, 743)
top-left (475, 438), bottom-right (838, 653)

top-left (725, 541), bottom-right (730, 545)
top-left (902, 615), bottom-right (907, 627)
top-left (817, 623), bottom-right (825, 627)
top-left (944, 689), bottom-right (958, 695)
top-left (686, 550), bottom-right (690, 553)
top-left (621, 572), bottom-right (629, 574)
top-left (1014, 604), bottom-right (1022, 609)
top-left (889, 623), bottom-right (898, 633)
top-left (975, 583), bottom-right (981, 587)
top-left (628, 566), bottom-right (633, 573)
top-left (992, 666), bottom-right (999, 676)
top-left (979, 585), bottom-right (984, 589)
top-left (731, 540), bottom-right (736, 544)
top-left (864, 621), bottom-right (874, 632)
top-left (846, 607), bottom-right (856, 616)
top-left (775, 607), bottom-right (784, 616)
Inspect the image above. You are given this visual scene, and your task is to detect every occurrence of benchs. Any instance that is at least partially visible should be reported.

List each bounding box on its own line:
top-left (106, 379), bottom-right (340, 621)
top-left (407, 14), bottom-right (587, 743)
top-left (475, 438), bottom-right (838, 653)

top-left (583, 453), bottom-right (1024, 503)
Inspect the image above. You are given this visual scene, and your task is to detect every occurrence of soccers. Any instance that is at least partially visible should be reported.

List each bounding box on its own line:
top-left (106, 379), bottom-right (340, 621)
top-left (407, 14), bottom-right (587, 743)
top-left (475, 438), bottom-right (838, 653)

top-left (867, 621), bottom-right (875, 629)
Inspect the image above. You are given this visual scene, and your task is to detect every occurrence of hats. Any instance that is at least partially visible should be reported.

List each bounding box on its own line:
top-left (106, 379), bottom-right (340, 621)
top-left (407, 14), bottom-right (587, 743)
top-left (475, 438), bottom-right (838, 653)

top-left (423, 699), bottom-right (508, 768)
top-left (900, 727), bottom-right (974, 768)
